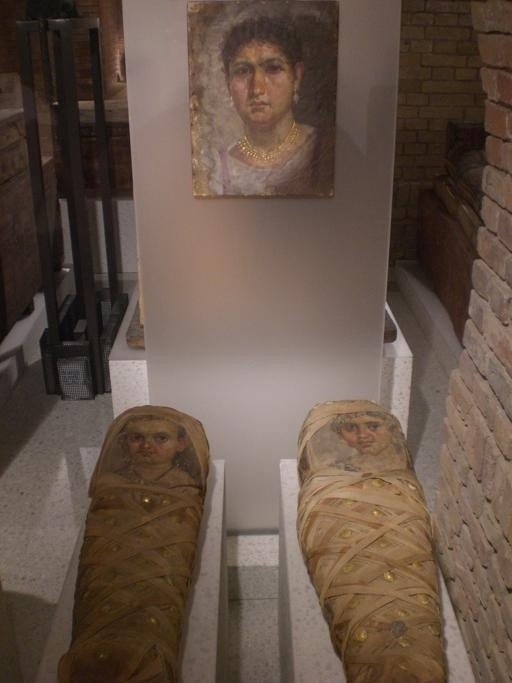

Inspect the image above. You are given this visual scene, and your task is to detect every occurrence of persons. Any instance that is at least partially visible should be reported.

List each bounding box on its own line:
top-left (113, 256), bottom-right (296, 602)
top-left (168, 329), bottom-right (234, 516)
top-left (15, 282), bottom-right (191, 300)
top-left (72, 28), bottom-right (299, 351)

top-left (201, 13), bottom-right (330, 192)
top-left (109, 413), bottom-right (195, 511)
top-left (325, 409), bottom-right (407, 480)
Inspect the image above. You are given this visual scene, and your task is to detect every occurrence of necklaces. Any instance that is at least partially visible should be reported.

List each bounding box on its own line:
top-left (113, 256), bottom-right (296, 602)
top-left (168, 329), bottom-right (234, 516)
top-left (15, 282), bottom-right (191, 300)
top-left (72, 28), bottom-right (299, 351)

top-left (135, 465), bottom-right (175, 503)
top-left (238, 119), bottom-right (300, 162)
